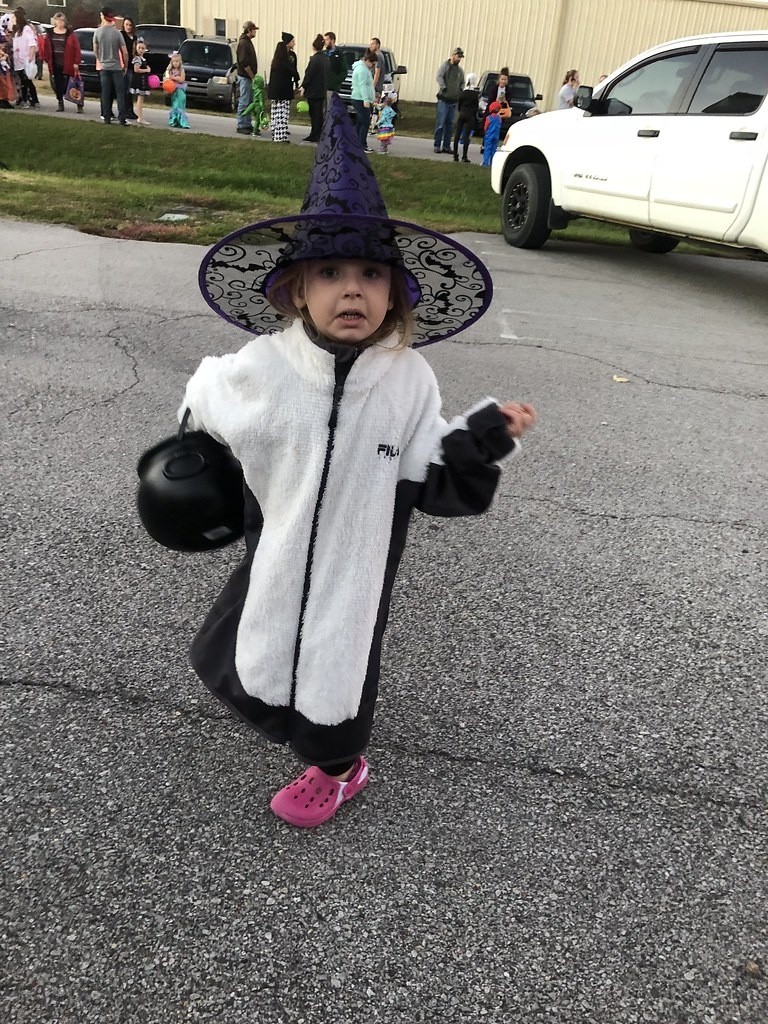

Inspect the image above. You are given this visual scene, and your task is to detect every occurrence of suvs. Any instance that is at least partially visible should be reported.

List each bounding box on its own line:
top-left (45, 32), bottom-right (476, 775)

top-left (472, 70), bottom-right (543, 139)
top-left (323, 42), bottom-right (407, 133)
top-left (134, 23), bottom-right (190, 90)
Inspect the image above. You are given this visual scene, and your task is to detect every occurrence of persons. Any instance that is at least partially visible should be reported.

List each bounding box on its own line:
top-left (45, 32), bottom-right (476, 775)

top-left (351, 37), bottom-right (398, 154)
top-left (433, 48), bottom-right (511, 166)
top-left (324, 32), bottom-right (348, 110)
top-left (242, 76), bottom-right (265, 136)
top-left (298, 34), bottom-right (332, 143)
top-left (282, 32), bottom-right (300, 91)
top-left (120, 15), bottom-right (138, 119)
top-left (166, 52), bottom-right (190, 128)
top-left (268, 42), bottom-right (296, 144)
top-left (180, 90), bottom-right (536, 827)
top-left (93, 7), bottom-right (133, 126)
top-left (130, 36), bottom-right (151, 125)
top-left (557, 70), bottom-right (607, 110)
top-left (235, 21), bottom-right (259, 135)
top-left (44, 11), bottom-right (84, 114)
top-left (0, 7), bottom-right (45, 110)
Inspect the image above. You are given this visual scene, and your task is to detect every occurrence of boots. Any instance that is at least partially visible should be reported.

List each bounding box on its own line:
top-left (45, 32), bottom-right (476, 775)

top-left (76, 104), bottom-right (83, 112)
top-left (55, 101), bottom-right (64, 111)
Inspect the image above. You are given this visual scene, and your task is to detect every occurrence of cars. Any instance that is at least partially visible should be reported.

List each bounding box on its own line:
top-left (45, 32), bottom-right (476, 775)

top-left (491, 28), bottom-right (767, 257)
top-left (162, 35), bottom-right (247, 113)
top-left (49, 27), bottom-right (133, 98)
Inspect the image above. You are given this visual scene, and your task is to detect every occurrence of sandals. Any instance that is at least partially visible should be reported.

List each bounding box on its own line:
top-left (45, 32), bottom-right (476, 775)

top-left (271, 755), bottom-right (368, 827)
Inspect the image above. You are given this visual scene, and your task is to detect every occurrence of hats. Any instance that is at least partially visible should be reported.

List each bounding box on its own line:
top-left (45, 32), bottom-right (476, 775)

top-left (197, 93), bottom-right (493, 349)
top-left (454, 47), bottom-right (464, 58)
top-left (243, 21), bottom-right (260, 30)
top-left (101, 7), bottom-right (116, 22)
top-left (282, 32), bottom-right (294, 44)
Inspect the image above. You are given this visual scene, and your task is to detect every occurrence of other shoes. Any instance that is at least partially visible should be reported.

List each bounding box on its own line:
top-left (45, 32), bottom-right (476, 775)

top-left (0, 97), bottom-right (40, 110)
top-left (434, 149), bottom-right (441, 153)
top-left (454, 157), bottom-right (459, 161)
top-left (375, 148), bottom-right (389, 154)
top-left (461, 158), bottom-right (471, 162)
top-left (364, 146), bottom-right (374, 154)
top-left (442, 147), bottom-right (454, 154)
top-left (236, 126), bottom-right (259, 134)
top-left (303, 134), bottom-right (319, 143)
top-left (99, 111), bottom-right (151, 125)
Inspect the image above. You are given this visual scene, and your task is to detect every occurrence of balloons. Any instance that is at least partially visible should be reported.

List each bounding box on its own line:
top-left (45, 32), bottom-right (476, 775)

top-left (148, 75), bottom-right (160, 89)
top-left (162, 80), bottom-right (177, 94)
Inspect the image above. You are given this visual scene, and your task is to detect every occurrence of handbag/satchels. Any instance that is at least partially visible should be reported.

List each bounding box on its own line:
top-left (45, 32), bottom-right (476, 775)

top-left (498, 101), bottom-right (512, 118)
top-left (65, 70), bottom-right (84, 106)
top-left (26, 57), bottom-right (38, 80)
